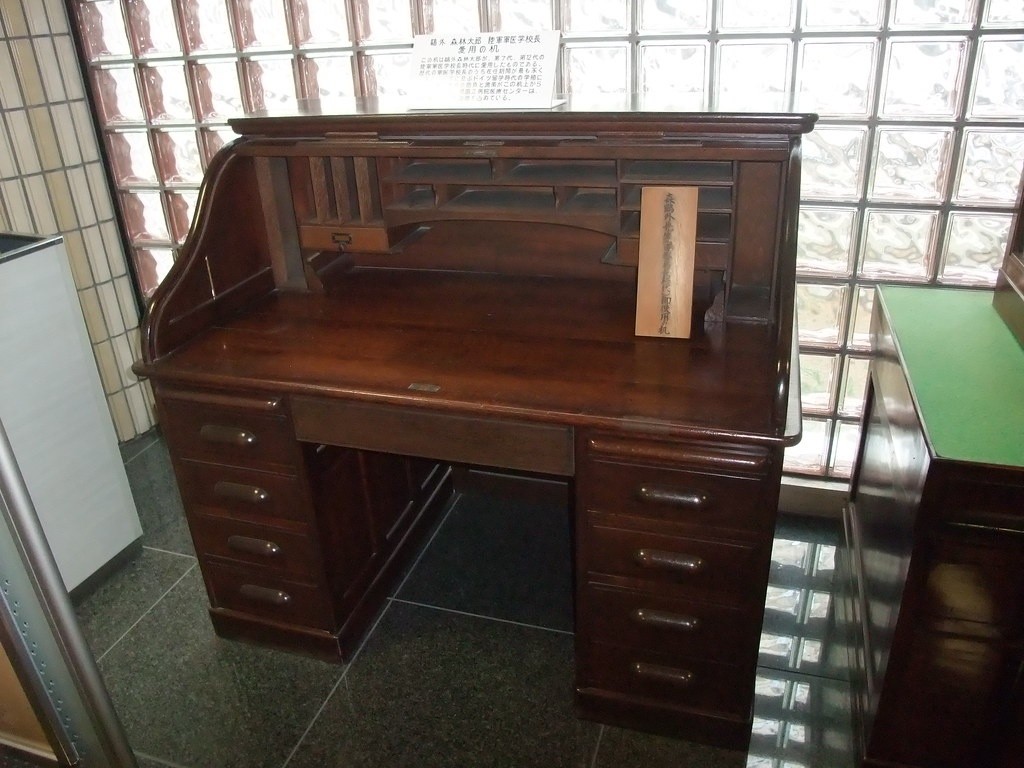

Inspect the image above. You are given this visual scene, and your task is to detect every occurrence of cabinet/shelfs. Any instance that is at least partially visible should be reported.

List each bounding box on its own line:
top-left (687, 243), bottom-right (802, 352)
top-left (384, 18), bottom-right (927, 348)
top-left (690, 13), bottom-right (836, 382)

top-left (131, 111), bottom-right (817, 749)
top-left (838, 165), bottom-right (1024, 767)
